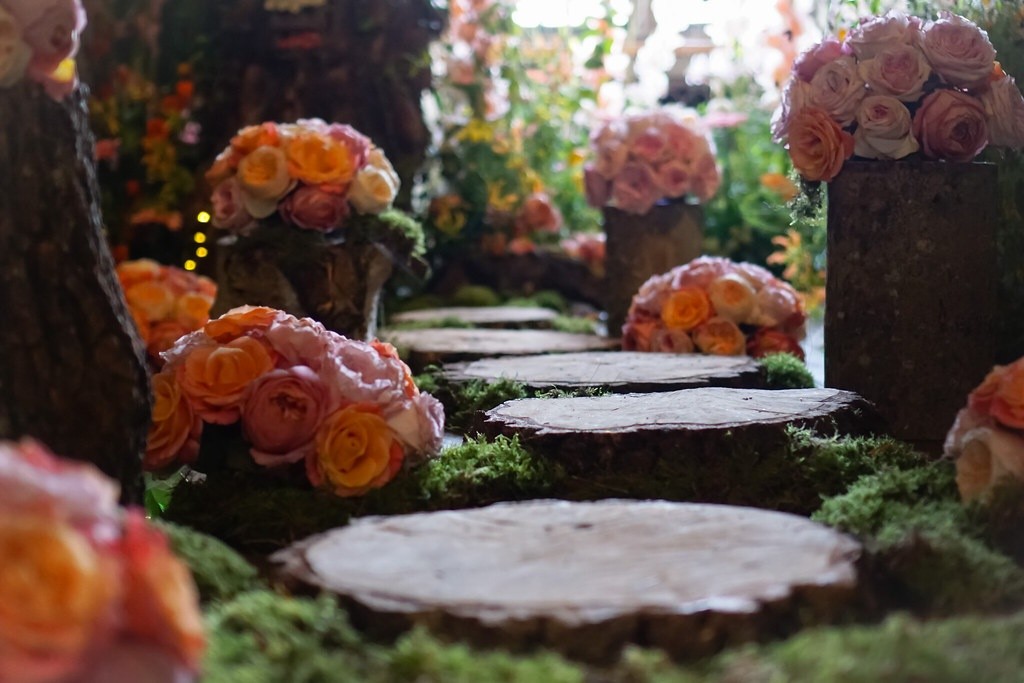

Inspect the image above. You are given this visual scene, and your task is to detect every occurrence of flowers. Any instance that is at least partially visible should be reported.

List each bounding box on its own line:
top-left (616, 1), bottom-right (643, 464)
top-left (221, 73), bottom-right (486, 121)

top-left (204, 115), bottom-right (401, 234)
top-left (585, 105), bottom-right (722, 216)
top-left (769, 10), bottom-right (1024, 183)
top-left (944, 357), bottom-right (1024, 508)
top-left (118, 257), bottom-right (215, 358)
top-left (141, 306), bottom-right (446, 499)
top-left (0, 0), bottom-right (88, 101)
top-left (0, 436), bottom-right (209, 683)
top-left (621, 252), bottom-right (810, 361)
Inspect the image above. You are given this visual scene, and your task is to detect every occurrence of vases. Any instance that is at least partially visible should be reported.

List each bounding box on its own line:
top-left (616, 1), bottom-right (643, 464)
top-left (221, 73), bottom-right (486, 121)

top-left (824, 159), bottom-right (1024, 456)
top-left (215, 234), bottom-right (391, 344)
top-left (0, 82), bottom-right (160, 509)
top-left (603, 201), bottom-right (707, 338)
